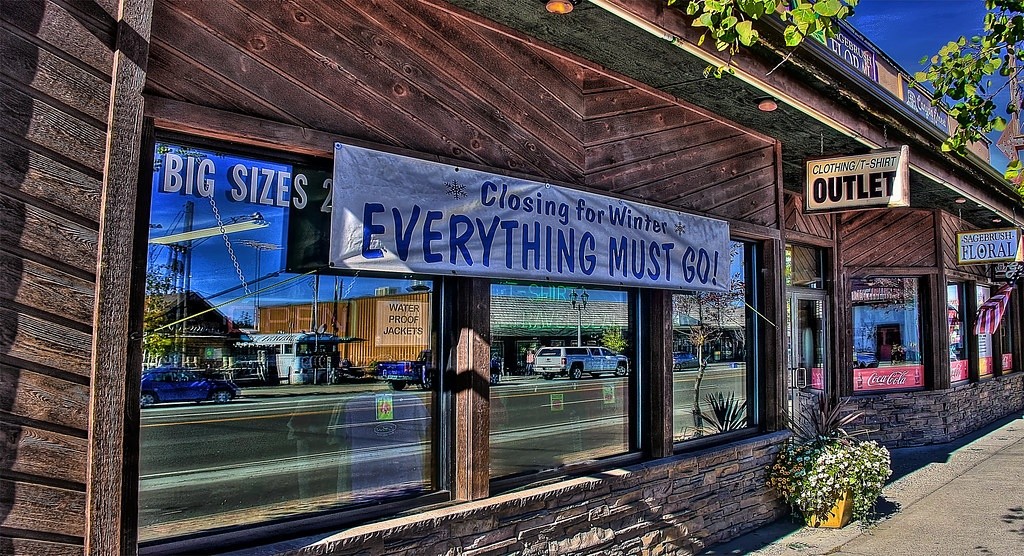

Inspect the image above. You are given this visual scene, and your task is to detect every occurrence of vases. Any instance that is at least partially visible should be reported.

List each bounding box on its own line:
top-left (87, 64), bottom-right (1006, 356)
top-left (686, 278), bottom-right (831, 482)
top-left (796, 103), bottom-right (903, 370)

top-left (803, 487), bottom-right (856, 528)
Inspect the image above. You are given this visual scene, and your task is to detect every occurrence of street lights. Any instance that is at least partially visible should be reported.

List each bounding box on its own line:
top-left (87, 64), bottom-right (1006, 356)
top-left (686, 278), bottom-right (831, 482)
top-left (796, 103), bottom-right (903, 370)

top-left (570, 288), bottom-right (589, 347)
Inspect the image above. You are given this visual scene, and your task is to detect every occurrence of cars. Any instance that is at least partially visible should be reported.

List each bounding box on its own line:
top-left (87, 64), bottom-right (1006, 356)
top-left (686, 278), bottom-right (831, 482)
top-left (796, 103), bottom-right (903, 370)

top-left (140, 365), bottom-right (241, 408)
top-left (673, 352), bottom-right (699, 369)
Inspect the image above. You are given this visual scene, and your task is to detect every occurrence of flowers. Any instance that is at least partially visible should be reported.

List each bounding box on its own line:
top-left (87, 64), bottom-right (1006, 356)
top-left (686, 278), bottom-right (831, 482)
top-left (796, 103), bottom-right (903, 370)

top-left (766, 439), bottom-right (893, 534)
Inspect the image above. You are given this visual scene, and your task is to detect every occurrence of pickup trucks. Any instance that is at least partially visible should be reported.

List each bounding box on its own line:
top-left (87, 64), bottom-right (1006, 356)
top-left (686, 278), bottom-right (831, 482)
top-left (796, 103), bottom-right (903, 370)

top-left (374, 350), bottom-right (433, 391)
top-left (532, 346), bottom-right (628, 380)
